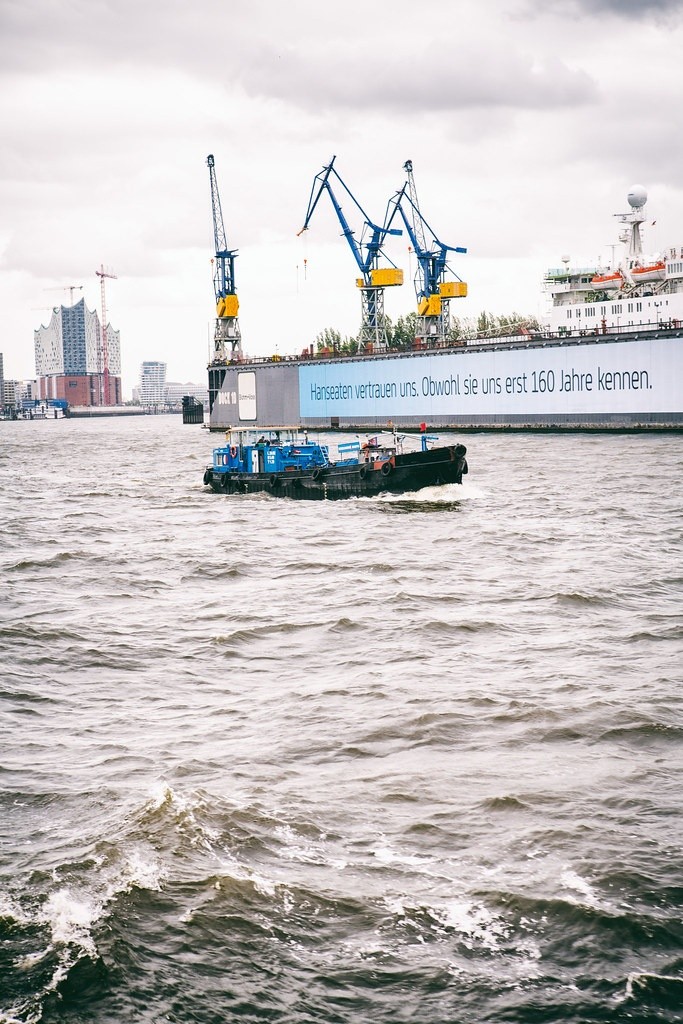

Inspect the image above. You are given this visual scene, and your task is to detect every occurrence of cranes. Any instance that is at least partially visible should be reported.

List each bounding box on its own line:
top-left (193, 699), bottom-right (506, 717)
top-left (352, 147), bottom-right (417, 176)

top-left (297, 154), bottom-right (468, 354)
top-left (207, 153), bottom-right (243, 364)
top-left (42, 286), bottom-right (83, 306)
top-left (95, 264), bottom-right (117, 406)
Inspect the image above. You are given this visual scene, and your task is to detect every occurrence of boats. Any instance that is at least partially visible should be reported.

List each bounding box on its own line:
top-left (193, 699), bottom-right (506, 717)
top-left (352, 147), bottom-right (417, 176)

top-left (203, 424), bottom-right (469, 500)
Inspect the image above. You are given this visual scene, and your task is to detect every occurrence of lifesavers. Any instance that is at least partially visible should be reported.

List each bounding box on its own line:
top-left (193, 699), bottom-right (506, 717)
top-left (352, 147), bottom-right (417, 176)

top-left (220, 474), bottom-right (229, 487)
top-left (204, 469), bottom-right (211, 485)
top-left (230, 447), bottom-right (237, 458)
top-left (359, 466), bottom-right (368, 480)
top-left (235, 481), bottom-right (243, 491)
top-left (454, 444), bottom-right (467, 458)
top-left (292, 477), bottom-right (300, 487)
top-left (270, 475), bottom-right (278, 487)
top-left (311, 468), bottom-right (319, 482)
top-left (381, 462), bottom-right (392, 476)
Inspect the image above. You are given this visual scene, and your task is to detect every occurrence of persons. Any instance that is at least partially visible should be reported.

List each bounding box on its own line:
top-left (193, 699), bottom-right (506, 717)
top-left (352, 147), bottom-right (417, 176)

top-left (259, 436), bottom-right (264, 443)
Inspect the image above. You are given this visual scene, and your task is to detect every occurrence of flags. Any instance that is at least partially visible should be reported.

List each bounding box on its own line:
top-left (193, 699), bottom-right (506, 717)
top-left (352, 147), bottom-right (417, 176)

top-left (420, 423), bottom-right (426, 431)
